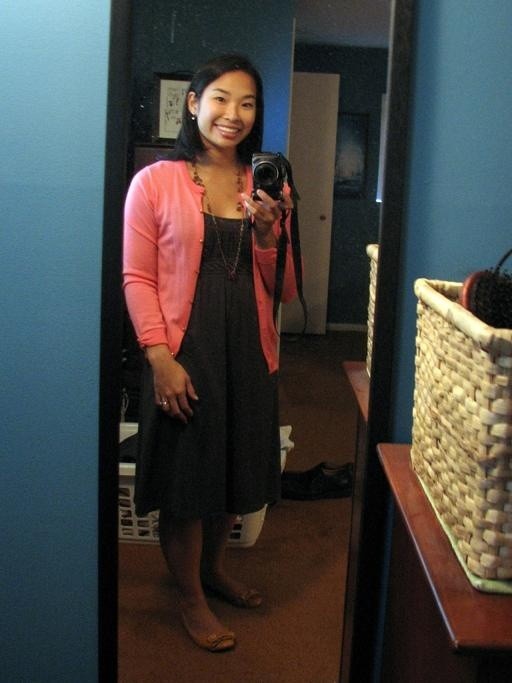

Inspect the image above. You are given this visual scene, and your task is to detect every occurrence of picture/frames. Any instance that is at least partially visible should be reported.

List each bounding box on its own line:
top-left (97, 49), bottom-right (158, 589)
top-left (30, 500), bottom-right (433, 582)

top-left (151, 72), bottom-right (193, 147)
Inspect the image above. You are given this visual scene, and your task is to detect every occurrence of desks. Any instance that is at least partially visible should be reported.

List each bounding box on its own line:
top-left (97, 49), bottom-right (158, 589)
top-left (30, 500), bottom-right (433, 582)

top-left (375, 442), bottom-right (510, 682)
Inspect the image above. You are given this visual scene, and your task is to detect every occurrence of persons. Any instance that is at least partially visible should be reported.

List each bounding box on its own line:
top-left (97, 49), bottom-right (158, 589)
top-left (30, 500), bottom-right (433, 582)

top-left (121, 48), bottom-right (307, 654)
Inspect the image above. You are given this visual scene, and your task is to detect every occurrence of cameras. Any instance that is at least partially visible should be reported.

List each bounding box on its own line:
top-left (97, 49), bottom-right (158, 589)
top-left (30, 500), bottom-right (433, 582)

top-left (252, 152), bottom-right (283, 201)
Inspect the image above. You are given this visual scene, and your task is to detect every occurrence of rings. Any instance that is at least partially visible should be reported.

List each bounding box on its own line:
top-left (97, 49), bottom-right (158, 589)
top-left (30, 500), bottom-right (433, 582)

top-left (158, 399), bottom-right (169, 407)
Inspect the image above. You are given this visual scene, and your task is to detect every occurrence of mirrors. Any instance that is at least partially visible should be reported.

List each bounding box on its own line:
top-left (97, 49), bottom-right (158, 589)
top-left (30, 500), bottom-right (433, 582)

top-left (97, 0), bottom-right (418, 683)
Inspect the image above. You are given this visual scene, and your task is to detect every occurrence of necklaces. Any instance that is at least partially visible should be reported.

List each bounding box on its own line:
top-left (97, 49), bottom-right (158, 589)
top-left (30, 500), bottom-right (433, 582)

top-left (188, 142), bottom-right (249, 282)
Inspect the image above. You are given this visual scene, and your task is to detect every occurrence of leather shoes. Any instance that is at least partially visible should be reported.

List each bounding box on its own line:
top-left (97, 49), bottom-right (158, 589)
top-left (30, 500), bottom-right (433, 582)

top-left (180, 607), bottom-right (235, 650)
top-left (280, 461), bottom-right (355, 499)
top-left (205, 574), bottom-right (263, 608)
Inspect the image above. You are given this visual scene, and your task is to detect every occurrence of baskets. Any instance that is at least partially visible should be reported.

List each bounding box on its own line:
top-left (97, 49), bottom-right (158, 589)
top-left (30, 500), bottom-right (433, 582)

top-left (365, 242), bottom-right (379, 376)
top-left (408, 277), bottom-right (512, 596)
top-left (118, 421), bottom-right (292, 549)
